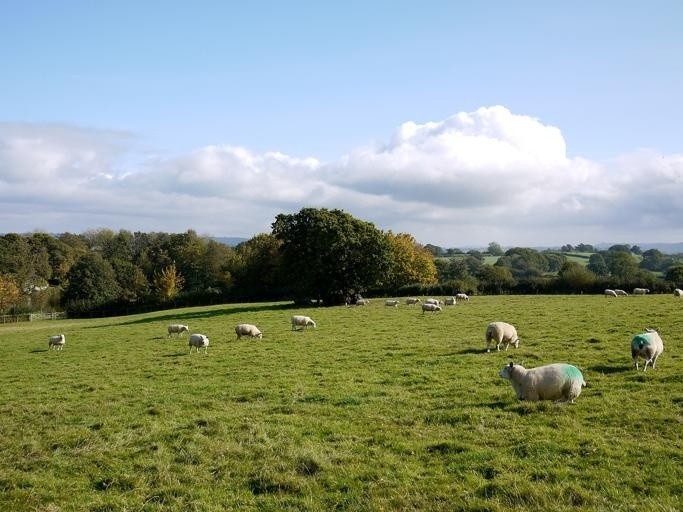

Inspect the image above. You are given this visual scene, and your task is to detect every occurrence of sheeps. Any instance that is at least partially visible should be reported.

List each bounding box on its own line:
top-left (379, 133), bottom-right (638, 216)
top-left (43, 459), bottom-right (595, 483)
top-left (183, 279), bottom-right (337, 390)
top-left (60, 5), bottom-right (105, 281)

top-left (188, 333), bottom-right (209, 355)
top-left (633, 288), bottom-right (650, 296)
top-left (234, 324), bottom-right (262, 340)
top-left (630, 326), bottom-right (664, 373)
top-left (456, 293), bottom-right (468, 301)
top-left (604, 289), bottom-right (628, 298)
top-left (167, 324), bottom-right (189, 338)
top-left (356, 299), bottom-right (369, 306)
top-left (291, 316), bottom-right (316, 331)
top-left (406, 296), bottom-right (456, 314)
top-left (486, 322), bottom-right (520, 353)
top-left (48, 333), bottom-right (65, 351)
top-left (674, 288), bottom-right (683, 298)
top-left (384, 300), bottom-right (400, 310)
top-left (498, 361), bottom-right (586, 403)
